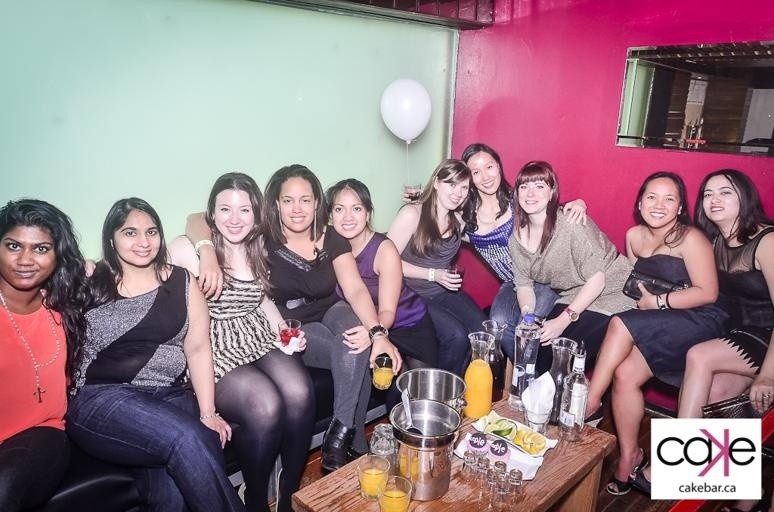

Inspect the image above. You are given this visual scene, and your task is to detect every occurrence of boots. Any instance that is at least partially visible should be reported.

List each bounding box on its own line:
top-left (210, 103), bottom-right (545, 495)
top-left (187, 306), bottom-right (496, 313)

top-left (321, 416), bottom-right (356, 471)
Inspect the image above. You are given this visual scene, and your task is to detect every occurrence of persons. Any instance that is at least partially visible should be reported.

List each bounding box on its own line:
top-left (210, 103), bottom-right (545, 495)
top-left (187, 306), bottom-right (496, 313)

top-left (385, 158), bottom-right (497, 384)
top-left (183, 163), bottom-right (404, 473)
top-left (65, 197), bottom-right (244, 512)
top-left (401, 143), bottom-right (587, 397)
top-left (165, 171), bottom-right (316, 512)
top-left (507, 161), bottom-right (638, 373)
top-left (579, 170), bottom-right (732, 500)
top-left (1, 198), bottom-right (97, 512)
top-left (630, 169), bottom-right (774, 512)
top-left (326, 179), bottom-right (439, 418)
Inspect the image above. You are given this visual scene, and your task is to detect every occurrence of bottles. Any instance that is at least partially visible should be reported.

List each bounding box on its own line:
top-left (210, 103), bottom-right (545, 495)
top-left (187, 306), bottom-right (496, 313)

top-left (686, 115), bottom-right (704, 141)
top-left (507, 314), bottom-right (541, 412)
top-left (555, 345), bottom-right (590, 442)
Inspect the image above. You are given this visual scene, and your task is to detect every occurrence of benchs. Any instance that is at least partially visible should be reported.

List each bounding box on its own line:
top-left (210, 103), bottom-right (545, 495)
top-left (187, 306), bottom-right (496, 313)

top-left (47, 369), bottom-right (389, 512)
top-left (633, 385), bottom-right (773, 453)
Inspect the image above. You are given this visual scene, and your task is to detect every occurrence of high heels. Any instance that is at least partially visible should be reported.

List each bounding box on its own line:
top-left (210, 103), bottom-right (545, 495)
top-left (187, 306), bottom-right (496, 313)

top-left (582, 402), bottom-right (604, 429)
top-left (626, 469), bottom-right (651, 495)
top-left (606, 448), bottom-right (648, 496)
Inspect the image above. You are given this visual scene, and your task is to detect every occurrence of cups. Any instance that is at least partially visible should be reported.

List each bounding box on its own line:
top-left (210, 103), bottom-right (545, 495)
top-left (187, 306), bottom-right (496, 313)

top-left (460, 450), bottom-right (523, 512)
top-left (392, 438), bottom-right (452, 503)
top-left (358, 455), bottom-right (389, 500)
top-left (377, 475), bottom-right (412, 510)
top-left (369, 423), bottom-right (398, 455)
top-left (278, 319), bottom-right (302, 345)
top-left (404, 183), bottom-right (421, 202)
top-left (371, 357), bottom-right (395, 392)
top-left (519, 407), bottom-right (553, 436)
top-left (447, 265), bottom-right (464, 289)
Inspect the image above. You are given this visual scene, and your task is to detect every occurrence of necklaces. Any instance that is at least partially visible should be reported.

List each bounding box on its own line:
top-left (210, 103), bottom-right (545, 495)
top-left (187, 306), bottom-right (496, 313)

top-left (1, 295), bottom-right (60, 404)
top-left (121, 281), bottom-right (161, 315)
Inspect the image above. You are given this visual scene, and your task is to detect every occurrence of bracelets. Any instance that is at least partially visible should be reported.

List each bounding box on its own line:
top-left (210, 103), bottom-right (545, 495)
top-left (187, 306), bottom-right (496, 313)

top-left (428, 268), bottom-right (435, 282)
top-left (368, 324), bottom-right (388, 340)
top-left (199, 413), bottom-right (218, 422)
top-left (657, 296), bottom-right (667, 309)
top-left (195, 240), bottom-right (216, 257)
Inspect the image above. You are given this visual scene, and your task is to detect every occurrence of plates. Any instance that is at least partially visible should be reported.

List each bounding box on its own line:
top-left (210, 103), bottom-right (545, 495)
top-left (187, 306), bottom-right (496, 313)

top-left (453, 433), bottom-right (545, 481)
top-left (471, 409), bottom-right (559, 457)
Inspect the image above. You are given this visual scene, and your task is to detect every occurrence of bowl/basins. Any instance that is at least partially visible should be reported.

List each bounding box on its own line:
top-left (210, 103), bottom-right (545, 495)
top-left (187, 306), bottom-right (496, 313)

top-left (388, 398), bottom-right (460, 447)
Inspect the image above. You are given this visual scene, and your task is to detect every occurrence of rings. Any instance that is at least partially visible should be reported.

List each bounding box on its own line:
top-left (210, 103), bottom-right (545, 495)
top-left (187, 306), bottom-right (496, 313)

top-left (762, 393), bottom-right (771, 401)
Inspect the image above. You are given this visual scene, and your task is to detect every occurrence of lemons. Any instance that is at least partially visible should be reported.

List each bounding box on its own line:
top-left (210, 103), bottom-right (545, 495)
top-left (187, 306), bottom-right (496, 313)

top-left (484, 419), bottom-right (546, 456)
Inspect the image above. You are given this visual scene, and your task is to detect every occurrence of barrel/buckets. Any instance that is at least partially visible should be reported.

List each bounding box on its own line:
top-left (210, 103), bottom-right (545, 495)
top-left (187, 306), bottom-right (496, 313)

top-left (389, 399), bottom-right (463, 502)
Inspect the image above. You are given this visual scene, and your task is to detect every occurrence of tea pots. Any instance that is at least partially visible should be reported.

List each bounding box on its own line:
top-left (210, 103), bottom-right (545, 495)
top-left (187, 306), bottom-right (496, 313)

top-left (481, 319), bottom-right (508, 402)
top-left (545, 336), bottom-right (576, 426)
top-left (462, 331), bottom-right (496, 417)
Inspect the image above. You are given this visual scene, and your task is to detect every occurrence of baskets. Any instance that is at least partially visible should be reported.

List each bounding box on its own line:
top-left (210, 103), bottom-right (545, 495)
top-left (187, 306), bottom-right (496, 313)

top-left (699, 393), bottom-right (763, 421)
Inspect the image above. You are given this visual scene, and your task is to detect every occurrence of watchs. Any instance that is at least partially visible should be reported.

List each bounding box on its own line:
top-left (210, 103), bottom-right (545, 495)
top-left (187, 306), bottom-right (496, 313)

top-left (565, 307), bottom-right (580, 323)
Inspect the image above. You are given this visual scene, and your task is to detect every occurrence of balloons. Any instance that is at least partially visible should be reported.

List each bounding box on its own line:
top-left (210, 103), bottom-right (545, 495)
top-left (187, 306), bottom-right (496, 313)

top-left (380, 79), bottom-right (432, 143)
